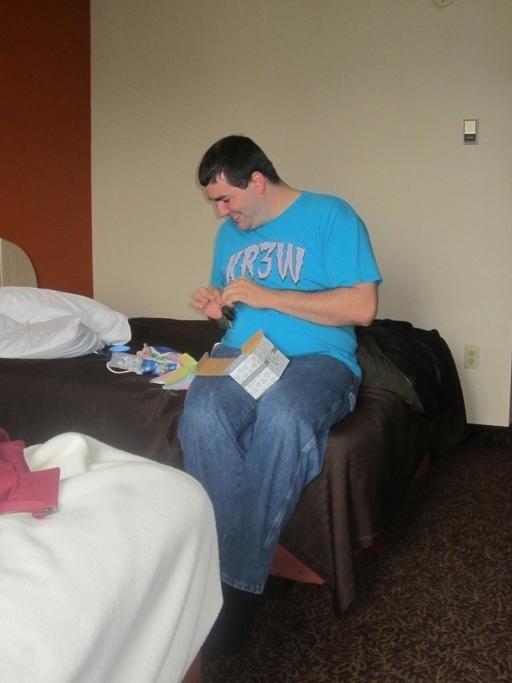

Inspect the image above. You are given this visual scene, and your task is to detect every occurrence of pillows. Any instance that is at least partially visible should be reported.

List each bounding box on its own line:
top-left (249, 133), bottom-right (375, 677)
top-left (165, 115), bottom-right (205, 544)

top-left (0, 313), bottom-right (105, 361)
top-left (0, 283), bottom-right (131, 347)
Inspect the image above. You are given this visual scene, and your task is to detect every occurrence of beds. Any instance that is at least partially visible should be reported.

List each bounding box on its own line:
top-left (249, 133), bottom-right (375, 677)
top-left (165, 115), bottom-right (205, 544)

top-left (0, 240), bottom-right (465, 612)
top-left (0, 431), bottom-right (224, 683)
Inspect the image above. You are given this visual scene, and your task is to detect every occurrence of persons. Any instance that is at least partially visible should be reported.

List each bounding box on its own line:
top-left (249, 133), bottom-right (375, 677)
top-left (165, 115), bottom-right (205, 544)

top-left (176, 134), bottom-right (384, 655)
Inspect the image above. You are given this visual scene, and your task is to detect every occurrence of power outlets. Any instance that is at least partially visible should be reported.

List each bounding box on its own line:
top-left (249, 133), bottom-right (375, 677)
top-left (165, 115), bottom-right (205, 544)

top-left (465, 344), bottom-right (479, 370)
